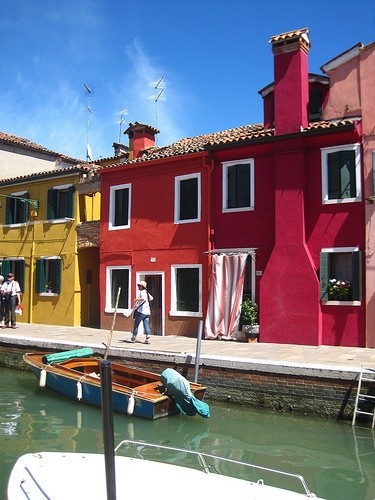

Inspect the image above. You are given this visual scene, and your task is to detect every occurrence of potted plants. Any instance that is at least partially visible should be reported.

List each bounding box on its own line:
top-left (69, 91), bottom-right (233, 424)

top-left (239, 298), bottom-right (259, 343)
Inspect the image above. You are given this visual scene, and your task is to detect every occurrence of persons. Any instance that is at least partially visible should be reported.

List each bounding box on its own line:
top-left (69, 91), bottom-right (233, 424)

top-left (0, 275), bottom-right (6, 326)
top-left (0, 273), bottom-right (21, 329)
top-left (126, 281), bottom-right (153, 344)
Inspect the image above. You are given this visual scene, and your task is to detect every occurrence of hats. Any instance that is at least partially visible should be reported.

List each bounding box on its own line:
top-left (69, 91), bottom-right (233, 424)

top-left (8, 273), bottom-right (14, 277)
top-left (137, 281), bottom-right (147, 290)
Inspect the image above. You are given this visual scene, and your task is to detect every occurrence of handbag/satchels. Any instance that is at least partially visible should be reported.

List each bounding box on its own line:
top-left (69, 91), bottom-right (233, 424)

top-left (133, 309), bottom-right (137, 319)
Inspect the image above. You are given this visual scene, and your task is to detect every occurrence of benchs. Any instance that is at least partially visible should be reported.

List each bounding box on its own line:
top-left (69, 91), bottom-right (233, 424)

top-left (112, 374), bottom-right (149, 388)
top-left (62, 361), bottom-right (98, 368)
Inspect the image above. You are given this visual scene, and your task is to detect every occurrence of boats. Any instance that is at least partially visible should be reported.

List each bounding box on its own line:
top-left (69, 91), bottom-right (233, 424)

top-left (22, 286), bottom-right (211, 421)
top-left (6, 358), bottom-right (328, 500)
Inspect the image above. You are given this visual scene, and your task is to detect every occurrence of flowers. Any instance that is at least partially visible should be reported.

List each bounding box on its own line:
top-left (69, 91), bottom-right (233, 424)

top-left (46, 280), bottom-right (54, 289)
top-left (327, 278), bottom-right (351, 297)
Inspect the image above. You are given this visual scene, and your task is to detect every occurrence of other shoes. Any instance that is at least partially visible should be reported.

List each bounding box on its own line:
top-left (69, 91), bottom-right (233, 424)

top-left (2, 324), bottom-right (9, 329)
top-left (126, 338), bottom-right (135, 342)
top-left (142, 340), bottom-right (150, 344)
top-left (12, 325), bottom-right (16, 329)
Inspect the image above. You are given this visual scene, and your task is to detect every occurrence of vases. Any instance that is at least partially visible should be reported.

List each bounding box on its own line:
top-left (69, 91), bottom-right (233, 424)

top-left (47, 288), bottom-right (52, 293)
